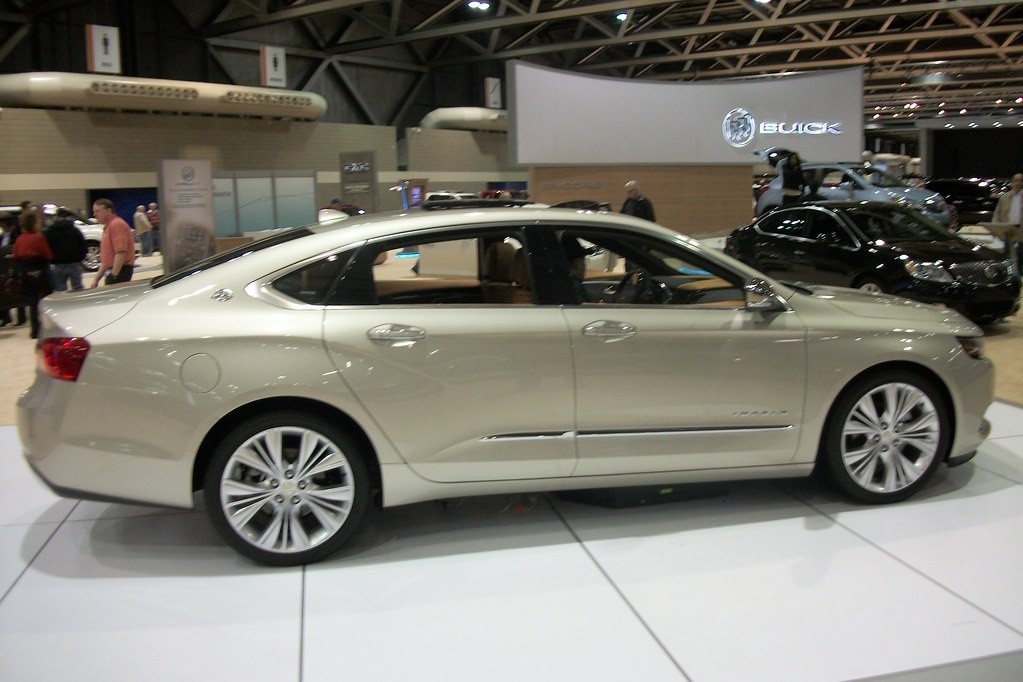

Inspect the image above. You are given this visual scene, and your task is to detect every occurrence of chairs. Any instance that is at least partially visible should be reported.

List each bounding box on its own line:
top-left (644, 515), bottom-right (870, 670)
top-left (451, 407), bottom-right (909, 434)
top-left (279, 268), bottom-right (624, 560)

top-left (481, 244), bottom-right (533, 304)
top-left (841, 174), bottom-right (855, 184)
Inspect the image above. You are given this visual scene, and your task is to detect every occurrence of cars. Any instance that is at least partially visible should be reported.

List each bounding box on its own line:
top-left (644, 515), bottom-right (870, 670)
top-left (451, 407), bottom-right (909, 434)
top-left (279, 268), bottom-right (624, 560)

top-left (480, 188), bottom-right (515, 201)
top-left (725, 200), bottom-right (1022, 329)
top-left (0, 207), bottom-right (145, 272)
top-left (14, 205), bottom-right (998, 567)
top-left (902, 174), bottom-right (1016, 215)
top-left (424, 190), bottom-right (475, 203)
top-left (749, 144), bottom-right (954, 235)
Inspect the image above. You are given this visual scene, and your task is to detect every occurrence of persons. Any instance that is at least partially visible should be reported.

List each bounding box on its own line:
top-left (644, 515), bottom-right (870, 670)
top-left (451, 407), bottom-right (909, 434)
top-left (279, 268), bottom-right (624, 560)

top-left (992, 171), bottom-right (1023, 277)
top-left (320, 180), bottom-right (655, 306)
top-left (0, 198), bottom-right (160, 338)
top-left (782, 152), bottom-right (807, 205)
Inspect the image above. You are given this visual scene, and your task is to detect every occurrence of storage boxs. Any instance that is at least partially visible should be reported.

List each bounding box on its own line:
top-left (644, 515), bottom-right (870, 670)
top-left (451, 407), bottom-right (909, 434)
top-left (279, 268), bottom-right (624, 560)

top-left (216, 237), bottom-right (253, 253)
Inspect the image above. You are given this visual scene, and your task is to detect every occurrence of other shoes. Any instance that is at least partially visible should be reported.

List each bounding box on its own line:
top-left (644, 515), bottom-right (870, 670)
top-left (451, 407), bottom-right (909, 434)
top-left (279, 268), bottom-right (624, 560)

top-left (15, 319), bottom-right (26, 326)
top-left (0, 319), bottom-right (12, 327)
top-left (32, 330), bottom-right (39, 338)
